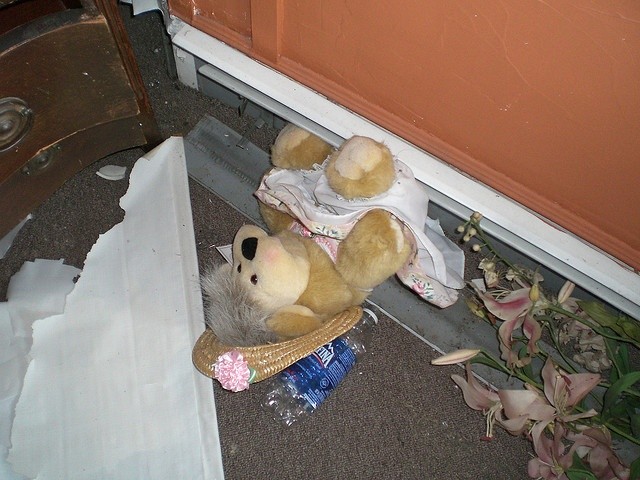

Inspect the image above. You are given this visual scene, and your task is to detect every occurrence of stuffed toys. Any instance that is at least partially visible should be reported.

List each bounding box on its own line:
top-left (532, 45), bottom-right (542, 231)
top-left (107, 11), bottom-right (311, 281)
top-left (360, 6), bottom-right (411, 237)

top-left (191, 117), bottom-right (429, 382)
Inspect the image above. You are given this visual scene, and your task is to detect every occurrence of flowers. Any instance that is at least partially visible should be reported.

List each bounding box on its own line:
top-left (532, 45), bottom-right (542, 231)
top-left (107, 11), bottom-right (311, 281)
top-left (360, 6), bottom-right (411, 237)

top-left (429, 210), bottom-right (640, 480)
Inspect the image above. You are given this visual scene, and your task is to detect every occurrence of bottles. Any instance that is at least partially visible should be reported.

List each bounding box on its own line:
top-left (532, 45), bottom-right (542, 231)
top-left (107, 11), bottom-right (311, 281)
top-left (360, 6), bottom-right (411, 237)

top-left (260, 305), bottom-right (380, 427)
